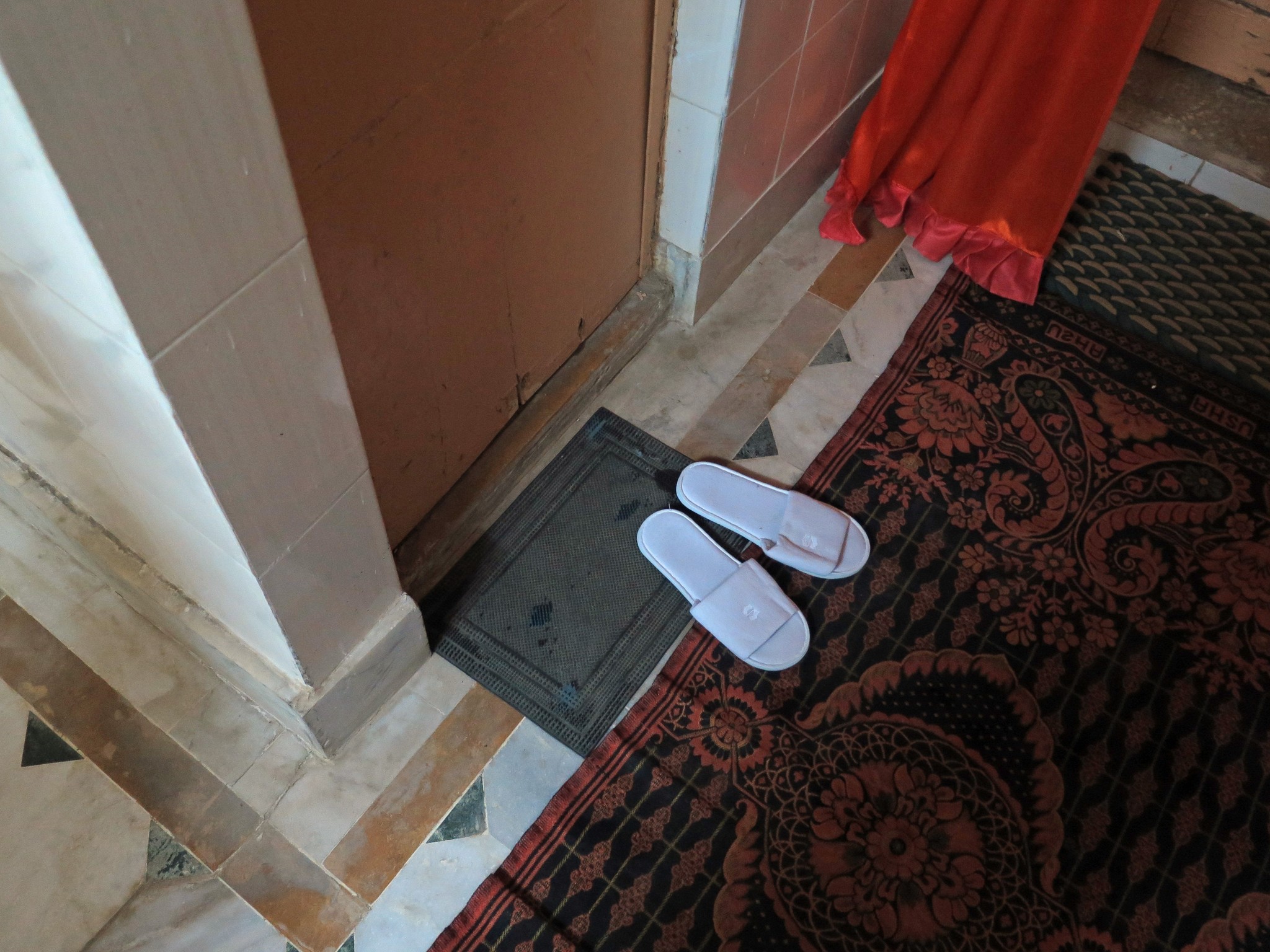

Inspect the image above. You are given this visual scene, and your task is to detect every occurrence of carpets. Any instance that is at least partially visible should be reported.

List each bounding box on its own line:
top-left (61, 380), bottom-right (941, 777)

top-left (430, 146), bottom-right (1270, 952)
top-left (418, 409), bottom-right (752, 761)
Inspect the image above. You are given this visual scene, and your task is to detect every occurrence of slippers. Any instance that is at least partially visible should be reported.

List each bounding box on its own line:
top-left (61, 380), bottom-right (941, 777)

top-left (676, 461), bottom-right (871, 579)
top-left (637, 509), bottom-right (811, 672)
